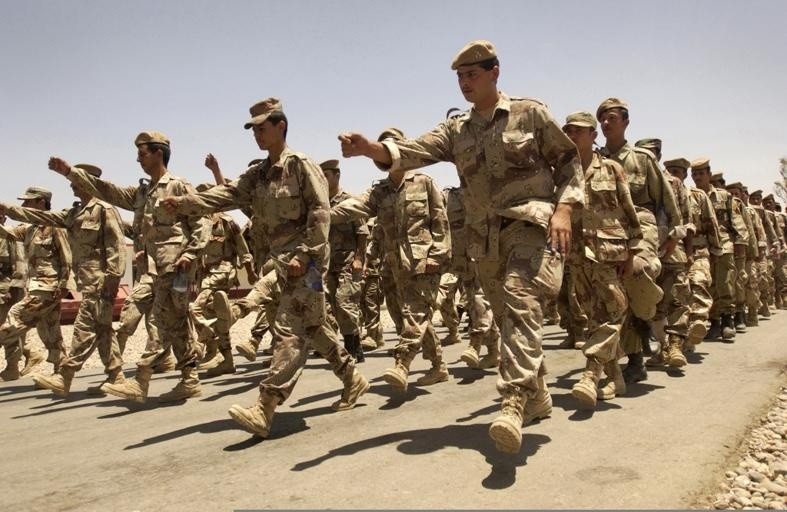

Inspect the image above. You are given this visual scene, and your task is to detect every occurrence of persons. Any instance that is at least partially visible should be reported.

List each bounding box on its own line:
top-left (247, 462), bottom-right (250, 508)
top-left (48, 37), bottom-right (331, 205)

top-left (0, 39), bottom-right (787, 456)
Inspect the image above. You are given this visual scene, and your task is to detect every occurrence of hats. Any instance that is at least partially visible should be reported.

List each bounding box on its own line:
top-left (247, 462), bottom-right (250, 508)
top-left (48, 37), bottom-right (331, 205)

top-left (596, 98), bottom-right (628, 121)
top-left (664, 158), bottom-right (781, 208)
top-left (562, 112), bottom-right (597, 133)
top-left (196, 184), bottom-right (215, 192)
top-left (320, 160), bottom-right (341, 170)
top-left (244, 98), bottom-right (283, 129)
top-left (135, 131), bottom-right (170, 147)
top-left (16, 188), bottom-right (52, 200)
top-left (74, 165), bottom-right (102, 177)
top-left (378, 128), bottom-right (405, 141)
top-left (451, 41), bottom-right (498, 70)
top-left (635, 139), bottom-right (661, 153)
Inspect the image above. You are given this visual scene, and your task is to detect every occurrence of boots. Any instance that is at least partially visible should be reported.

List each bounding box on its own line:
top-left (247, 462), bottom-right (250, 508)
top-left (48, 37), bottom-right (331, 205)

top-left (523, 376), bottom-right (553, 426)
top-left (32, 367), bottom-right (74, 398)
top-left (100, 367), bottom-right (154, 404)
top-left (331, 367), bottom-right (370, 411)
top-left (0, 343), bottom-right (45, 381)
top-left (160, 367), bottom-right (204, 402)
top-left (384, 306), bottom-right (502, 395)
top-left (213, 290), bottom-right (242, 333)
top-left (344, 326), bottom-right (384, 363)
top-left (704, 295), bottom-right (787, 339)
top-left (228, 392), bottom-right (280, 438)
top-left (153, 336), bottom-right (273, 376)
top-left (559, 320), bottom-right (707, 406)
top-left (88, 367), bottom-right (125, 394)
top-left (490, 394), bottom-right (527, 454)
